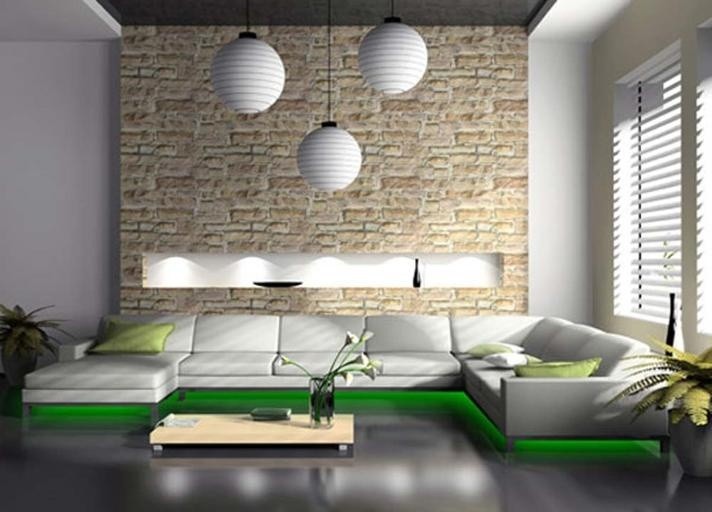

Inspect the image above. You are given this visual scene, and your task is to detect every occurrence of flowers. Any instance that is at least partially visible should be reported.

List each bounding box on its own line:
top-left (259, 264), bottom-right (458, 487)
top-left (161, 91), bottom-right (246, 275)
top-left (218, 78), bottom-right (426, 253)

top-left (271, 330), bottom-right (383, 423)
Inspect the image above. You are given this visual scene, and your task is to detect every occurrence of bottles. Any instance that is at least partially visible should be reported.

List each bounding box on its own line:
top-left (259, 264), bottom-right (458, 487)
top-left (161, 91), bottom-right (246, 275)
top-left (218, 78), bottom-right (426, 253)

top-left (413, 258), bottom-right (421, 287)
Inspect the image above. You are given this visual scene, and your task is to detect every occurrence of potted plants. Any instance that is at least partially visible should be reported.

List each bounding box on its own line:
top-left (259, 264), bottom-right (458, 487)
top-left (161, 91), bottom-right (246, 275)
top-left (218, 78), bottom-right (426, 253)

top-left (603, 338), bottom-right (712, 478)
top-left (0, 303), bottom-right (82, 417)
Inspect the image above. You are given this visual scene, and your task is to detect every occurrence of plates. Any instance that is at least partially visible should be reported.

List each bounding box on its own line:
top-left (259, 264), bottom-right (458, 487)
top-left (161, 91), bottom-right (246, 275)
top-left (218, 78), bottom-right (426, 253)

top-left (253, 282), bottom-right (302, 287)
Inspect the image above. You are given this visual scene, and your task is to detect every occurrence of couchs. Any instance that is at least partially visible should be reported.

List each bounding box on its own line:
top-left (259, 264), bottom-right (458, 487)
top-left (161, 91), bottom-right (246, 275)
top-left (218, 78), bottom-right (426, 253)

top-left (22, 312), bottom-right (669, 439)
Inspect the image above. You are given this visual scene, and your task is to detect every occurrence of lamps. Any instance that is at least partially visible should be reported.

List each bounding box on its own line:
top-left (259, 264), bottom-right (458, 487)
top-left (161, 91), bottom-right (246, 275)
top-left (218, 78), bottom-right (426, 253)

top-left (358, 0), bottom-right (428, 97)
top-left (210, 0), bottom-right (285, 114)
top-left (297, 1), bottom-right (361, 193)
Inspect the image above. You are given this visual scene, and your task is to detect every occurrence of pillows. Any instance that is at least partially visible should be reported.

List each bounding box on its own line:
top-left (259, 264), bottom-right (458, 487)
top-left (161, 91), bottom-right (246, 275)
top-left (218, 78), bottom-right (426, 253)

top-left (86, 315), bottom-right (173, 355)
top-left (466, 339), bottom-right (525, 357)
top-left (513, 357), bottom-right (604, 378)
top-left (483, 353), bottom-right (543, 368)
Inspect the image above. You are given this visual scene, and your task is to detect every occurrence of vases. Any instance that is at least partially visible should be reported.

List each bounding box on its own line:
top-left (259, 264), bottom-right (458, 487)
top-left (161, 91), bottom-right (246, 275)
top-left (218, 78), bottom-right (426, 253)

top-left (307, 376), bottom-right (335, 429)
